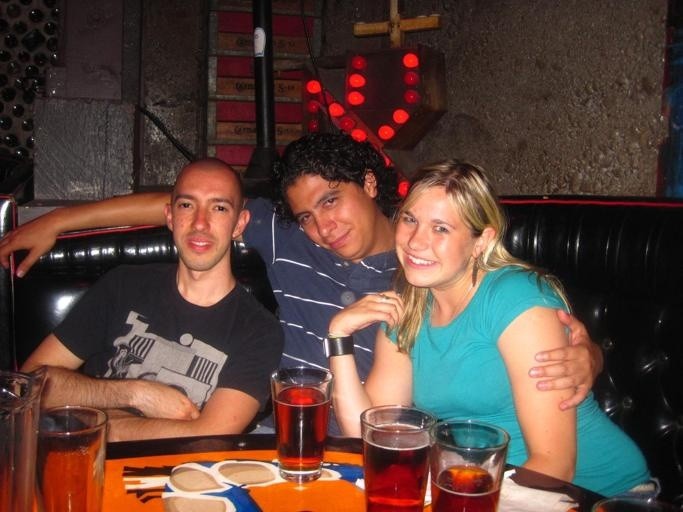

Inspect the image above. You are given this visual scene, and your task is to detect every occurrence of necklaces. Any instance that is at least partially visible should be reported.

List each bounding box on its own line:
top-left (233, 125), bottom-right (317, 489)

top-left (440, 283), bottom-right (480, 323)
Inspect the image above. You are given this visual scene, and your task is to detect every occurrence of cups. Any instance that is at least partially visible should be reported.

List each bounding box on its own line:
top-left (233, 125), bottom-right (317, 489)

top-left (270, 366), bottom-right (333, 483)
top-left (359, 404), bottom-right (437, 512)
top-left (33, 405), bottom-right (107, 511)
top-left (428, 419), bottom-right (510, 512)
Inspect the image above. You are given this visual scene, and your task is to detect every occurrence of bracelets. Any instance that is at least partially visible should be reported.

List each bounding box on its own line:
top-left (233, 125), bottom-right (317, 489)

top-left (322, 336), bottom-right (355, 359)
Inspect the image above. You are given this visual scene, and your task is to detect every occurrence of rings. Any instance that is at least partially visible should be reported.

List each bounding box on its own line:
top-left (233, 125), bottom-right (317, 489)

top-left (379, 295), bottom-right (391, 304)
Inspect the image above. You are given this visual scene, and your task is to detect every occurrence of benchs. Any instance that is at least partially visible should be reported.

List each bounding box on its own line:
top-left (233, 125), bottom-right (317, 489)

top-left (5, 196), bottom-right (683, 508)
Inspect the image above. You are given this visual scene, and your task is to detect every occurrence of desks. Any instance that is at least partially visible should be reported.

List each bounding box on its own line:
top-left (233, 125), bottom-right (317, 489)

top-left (1, 436), bottom-right (646, 512)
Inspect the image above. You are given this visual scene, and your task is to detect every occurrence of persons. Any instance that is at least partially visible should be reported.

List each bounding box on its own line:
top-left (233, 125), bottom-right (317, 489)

top-left (320, 155), bottom-right (663, 510)
top-left (2, 154), bottom-right (286, 463)
top-left (2, 128), bottom-right (606, 456)
